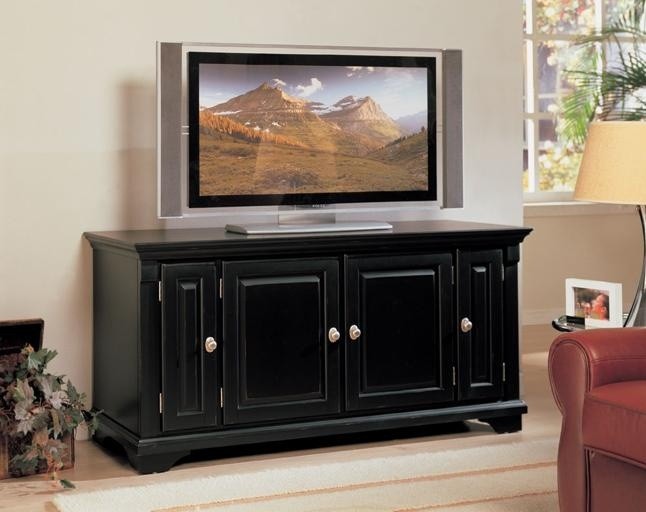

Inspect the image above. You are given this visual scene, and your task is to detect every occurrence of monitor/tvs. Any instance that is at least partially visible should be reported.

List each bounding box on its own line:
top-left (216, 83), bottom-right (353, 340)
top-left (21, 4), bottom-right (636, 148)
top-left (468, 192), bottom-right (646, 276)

top-left (156, 41), bottom-right (464, 234)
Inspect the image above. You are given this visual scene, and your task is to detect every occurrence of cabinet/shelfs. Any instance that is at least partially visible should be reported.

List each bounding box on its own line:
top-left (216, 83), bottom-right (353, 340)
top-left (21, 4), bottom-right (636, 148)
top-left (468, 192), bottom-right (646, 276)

top-left (80, 218), bottom-right (535, 477)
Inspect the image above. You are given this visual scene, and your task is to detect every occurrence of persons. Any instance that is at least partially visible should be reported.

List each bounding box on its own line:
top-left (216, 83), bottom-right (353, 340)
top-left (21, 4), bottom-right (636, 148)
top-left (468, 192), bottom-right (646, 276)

top-left (582, 293), bottom-right (609, 319)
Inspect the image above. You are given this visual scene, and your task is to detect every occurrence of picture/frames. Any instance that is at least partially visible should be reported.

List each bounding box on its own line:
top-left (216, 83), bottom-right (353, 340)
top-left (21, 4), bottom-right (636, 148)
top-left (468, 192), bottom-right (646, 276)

top-left (563, 276), bottom-right (624, 331)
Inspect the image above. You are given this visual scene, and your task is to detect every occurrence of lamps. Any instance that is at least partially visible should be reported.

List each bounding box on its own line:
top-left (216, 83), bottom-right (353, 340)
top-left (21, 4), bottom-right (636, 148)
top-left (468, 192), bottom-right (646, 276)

top-left (573, 115), bottom-right (645, 328)
